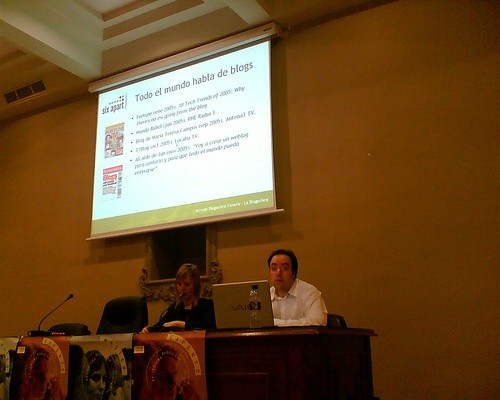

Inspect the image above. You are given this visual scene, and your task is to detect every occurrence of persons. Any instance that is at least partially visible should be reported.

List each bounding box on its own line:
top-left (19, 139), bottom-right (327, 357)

top-left (130, 337), bottom-right (202, 400)
top-left (138, 263), bottom-right (216, 333)
top-left (266, 250), bottom-right (328, 327)
top-left (68, 344), bottom-right (132, 400)
top-left (0, 343), bottom-right (65, 400)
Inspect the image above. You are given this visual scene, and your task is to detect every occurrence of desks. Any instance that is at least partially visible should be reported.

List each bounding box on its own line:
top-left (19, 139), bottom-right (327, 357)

top-left (0, 327), bottom-right (376, 400)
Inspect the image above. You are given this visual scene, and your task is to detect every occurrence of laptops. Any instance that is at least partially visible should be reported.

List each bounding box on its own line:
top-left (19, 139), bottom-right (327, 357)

top-left (212, 279), bottom-right (289, 330)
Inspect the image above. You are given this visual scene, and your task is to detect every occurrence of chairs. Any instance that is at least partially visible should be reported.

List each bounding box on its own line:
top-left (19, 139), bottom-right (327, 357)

top-left (97, 296), bottom-right (148, 334)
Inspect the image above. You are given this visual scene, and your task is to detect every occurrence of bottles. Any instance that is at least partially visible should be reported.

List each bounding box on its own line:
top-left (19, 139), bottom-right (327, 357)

top-left (249, 284), bottom-right (262, 329)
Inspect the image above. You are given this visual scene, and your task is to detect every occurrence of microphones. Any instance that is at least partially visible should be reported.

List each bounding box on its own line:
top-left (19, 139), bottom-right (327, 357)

top-left (30, 294), bottom-right (73, 337)
top-left (157, 291), bottom-right (185, 331)
